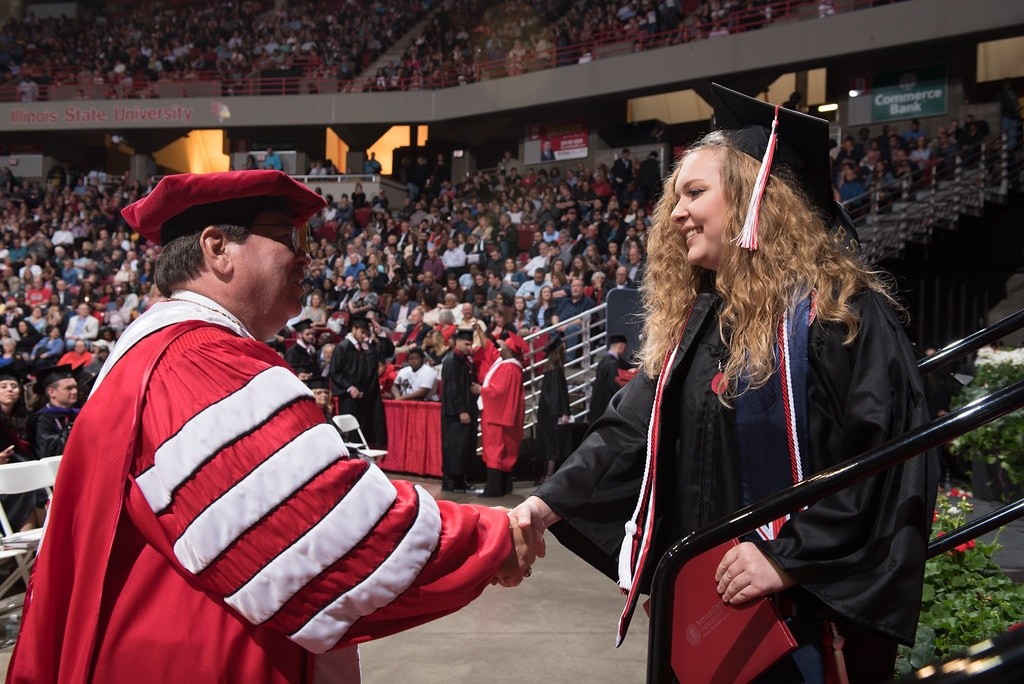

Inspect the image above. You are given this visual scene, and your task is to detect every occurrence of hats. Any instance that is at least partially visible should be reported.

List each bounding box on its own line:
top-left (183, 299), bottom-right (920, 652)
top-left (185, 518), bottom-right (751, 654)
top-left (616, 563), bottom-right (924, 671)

top-left (542, 333), bottom-right (564, 354)
top-left (504, 330), bottom-right (529, 356)
top-left (36, 363), bottom-right (74, 389)
top-left (609, 333), bottom-right (628, 344)
top-left (121, 169), bottom-right (328, 246)
top-left (709, 80), bottom-right (865, 256)
top-left (349, 315), bottom-right (371, 326)
top-left (456, 329), bottom-right (474, 342)
top-left (0, 361), bottom-right (21, 386)
top-left (292, 318), bottom-right (313, 332)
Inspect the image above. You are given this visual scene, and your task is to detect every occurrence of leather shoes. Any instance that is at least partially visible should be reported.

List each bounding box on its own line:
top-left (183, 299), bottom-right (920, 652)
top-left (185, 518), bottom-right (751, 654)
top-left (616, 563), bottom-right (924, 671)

top-left (441, 479), bottom-right (475, 493)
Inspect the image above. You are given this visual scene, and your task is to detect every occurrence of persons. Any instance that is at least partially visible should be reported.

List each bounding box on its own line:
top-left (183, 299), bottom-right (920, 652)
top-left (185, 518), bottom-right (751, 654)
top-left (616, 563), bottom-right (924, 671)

top-left (7, 168), bottom-right (546, 684)
top-left (0, 0), bottom-right (989, 581)
top-left (491, 82), bottom-right (940, 684)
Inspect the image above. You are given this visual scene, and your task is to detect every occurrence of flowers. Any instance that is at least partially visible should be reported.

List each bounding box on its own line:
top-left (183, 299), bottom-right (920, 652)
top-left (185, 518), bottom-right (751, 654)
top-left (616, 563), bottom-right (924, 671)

top-left (939, 344), bottom-right (1024, 503)
top-left (894, 485), bottom-right (1024, 675)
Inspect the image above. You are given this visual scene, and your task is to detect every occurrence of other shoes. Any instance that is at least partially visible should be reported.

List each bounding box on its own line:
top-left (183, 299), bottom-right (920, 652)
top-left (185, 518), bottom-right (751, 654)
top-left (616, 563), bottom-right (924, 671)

top-left (478, 492), bottom-right (496, 499)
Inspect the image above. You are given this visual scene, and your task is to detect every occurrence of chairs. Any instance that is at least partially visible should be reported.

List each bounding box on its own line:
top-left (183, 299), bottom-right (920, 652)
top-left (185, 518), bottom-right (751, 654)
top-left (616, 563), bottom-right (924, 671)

top-left (0, 455), bottom-right (64, 616)
top-left (333, 413), bottom-right (389, 463)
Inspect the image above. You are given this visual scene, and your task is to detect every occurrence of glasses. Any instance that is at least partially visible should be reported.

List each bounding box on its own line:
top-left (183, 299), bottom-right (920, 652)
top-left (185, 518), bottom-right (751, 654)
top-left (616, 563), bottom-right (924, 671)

top-left (227, 222), bottom-right (313, 253)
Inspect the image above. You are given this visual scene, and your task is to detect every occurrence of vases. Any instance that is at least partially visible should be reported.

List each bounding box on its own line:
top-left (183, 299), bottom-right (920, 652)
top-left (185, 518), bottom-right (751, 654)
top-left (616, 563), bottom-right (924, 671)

top-left (972, 445), bottom-right (1018, 504)
